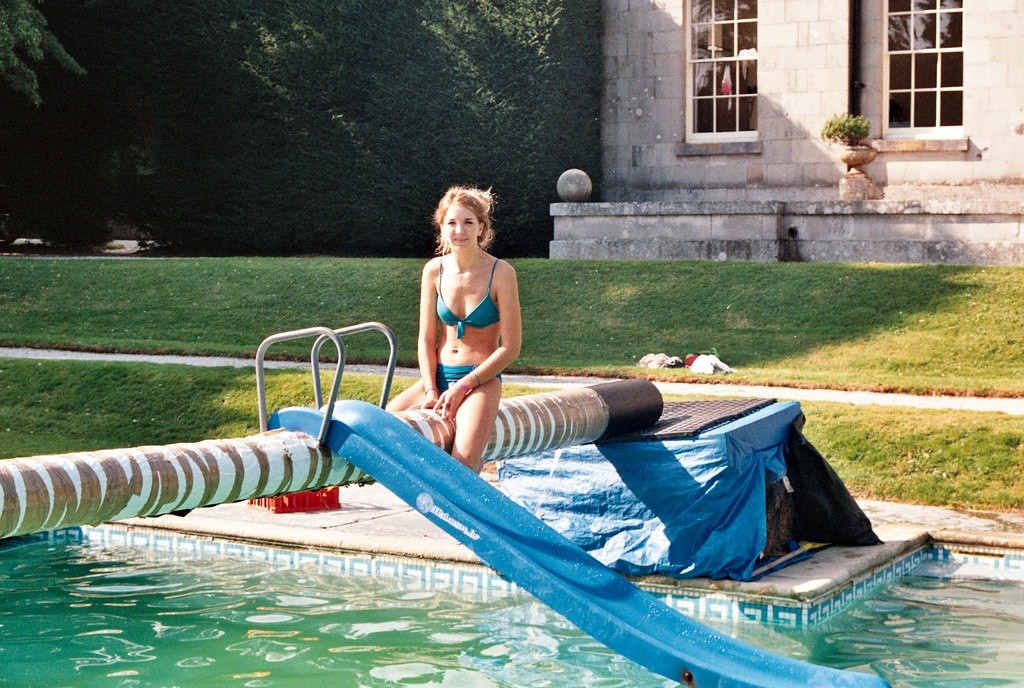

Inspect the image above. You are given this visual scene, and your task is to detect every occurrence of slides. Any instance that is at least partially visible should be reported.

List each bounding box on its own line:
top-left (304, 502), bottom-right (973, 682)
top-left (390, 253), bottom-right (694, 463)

top-left (267, 395), bottom-right (894, 688)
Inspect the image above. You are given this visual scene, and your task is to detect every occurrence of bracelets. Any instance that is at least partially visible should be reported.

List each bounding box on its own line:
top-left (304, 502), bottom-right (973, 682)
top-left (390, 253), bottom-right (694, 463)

top-left (457, 370), bottom-right (481, 392)
top-left (425, 388), bottom-right (441, 397)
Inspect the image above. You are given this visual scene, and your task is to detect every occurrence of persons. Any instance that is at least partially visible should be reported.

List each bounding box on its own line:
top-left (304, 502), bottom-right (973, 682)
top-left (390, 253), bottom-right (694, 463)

top-left (380, 187), bottom-right (521, 469)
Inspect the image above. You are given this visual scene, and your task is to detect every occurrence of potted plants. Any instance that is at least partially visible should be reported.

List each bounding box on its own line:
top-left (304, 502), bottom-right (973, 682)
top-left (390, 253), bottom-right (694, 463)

top-left (819, 112), bottom-right (877, 201)
